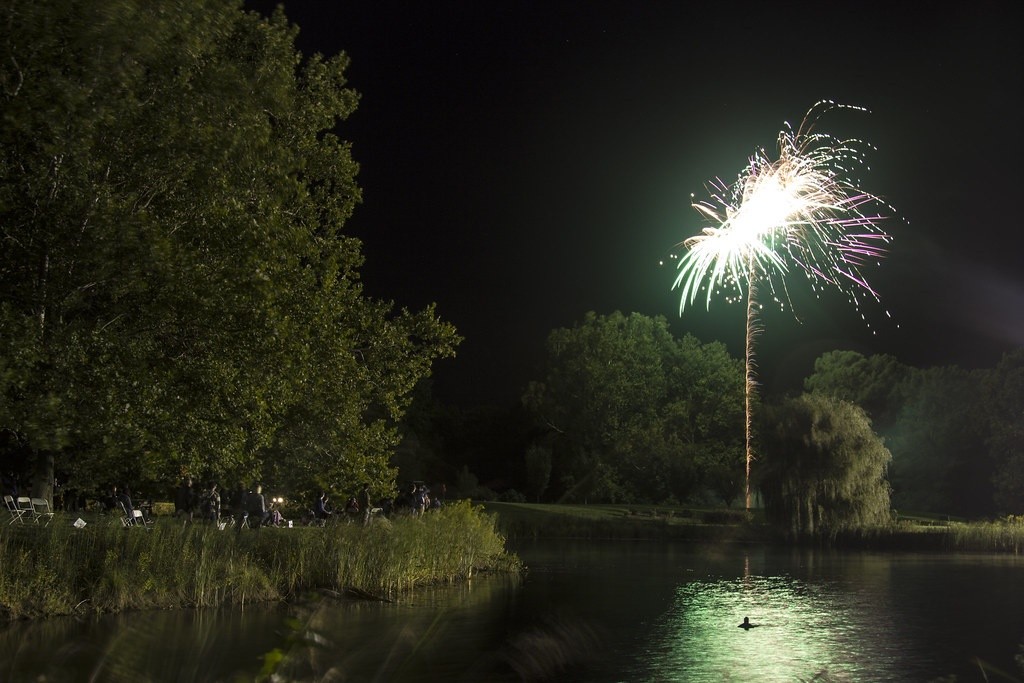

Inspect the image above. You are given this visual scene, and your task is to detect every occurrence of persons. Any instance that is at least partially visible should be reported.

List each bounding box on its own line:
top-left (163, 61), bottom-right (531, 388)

top-left (105, 488), bottom-right (154, 524)
top-left (78, 491), bottom-right (87, 511)
top-left (175, 477), bottom-right (194, 526)
top-left (315, 490), bottom-right (338, 520)
top-left (200, 481), bottom-right (222, 525)
top-left (345, 483), bottom-right (372, 528)
top-left (231, 481), bottom-right (286, 528)
top-left (405, 483), bottom-right (441, 513)
top-left (7, 471), bottom-right (17, 496)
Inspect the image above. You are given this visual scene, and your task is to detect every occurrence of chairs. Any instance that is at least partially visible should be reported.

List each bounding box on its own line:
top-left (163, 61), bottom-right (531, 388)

top-left (4, 494), bottom-right (27, 524)
top-left (132, 510), bottom-right (146, 526)
top-left (17, 496), bottom-right (34, 521)
top-left (32, 498), bottom-right (55, 527)
